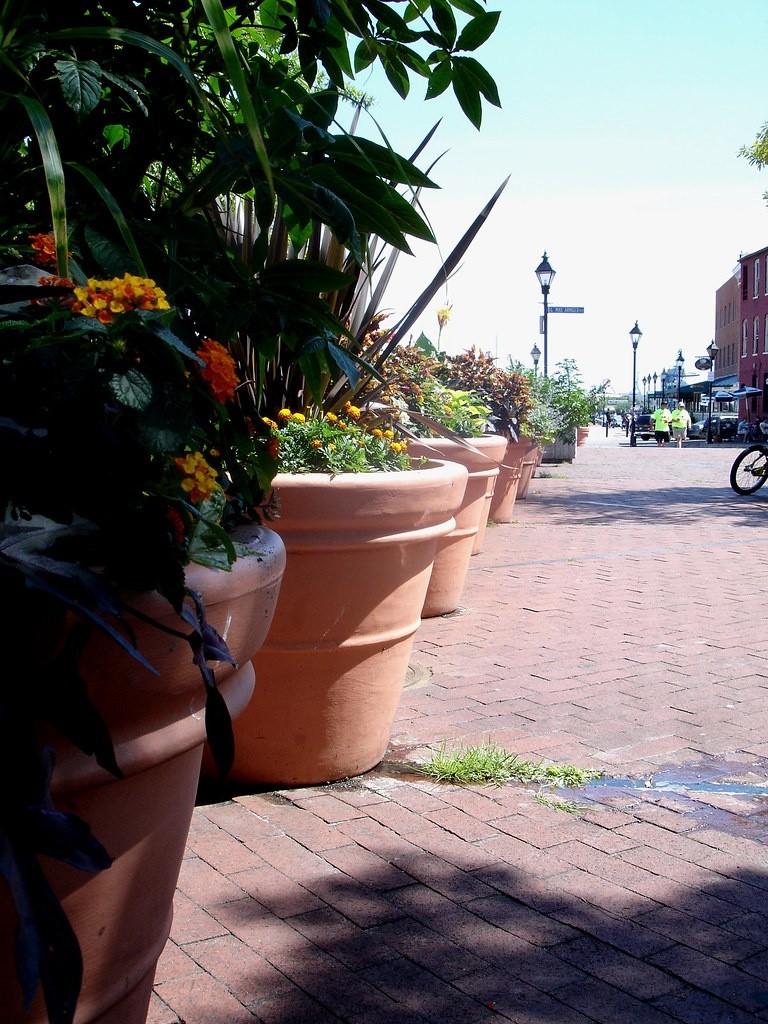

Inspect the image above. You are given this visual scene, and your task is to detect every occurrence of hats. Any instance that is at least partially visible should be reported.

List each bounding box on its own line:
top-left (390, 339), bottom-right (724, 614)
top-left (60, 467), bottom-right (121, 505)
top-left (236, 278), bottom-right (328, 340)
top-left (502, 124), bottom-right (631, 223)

top-left (678, 402), bottom-right (684, 406)
top-left (661, 401), bottom-right (669, 405)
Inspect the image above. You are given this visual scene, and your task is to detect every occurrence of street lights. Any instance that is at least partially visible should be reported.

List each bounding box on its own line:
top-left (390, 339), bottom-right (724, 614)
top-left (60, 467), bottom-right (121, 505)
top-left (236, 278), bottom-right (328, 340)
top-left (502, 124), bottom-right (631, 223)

top-left (674, 351), bottom-right (685, 407)
top-left (642, 369), bottom-right (668, 415)
top-left (706, 340), bottom-right (720, 444)
top-left (628, 321), bottom-right (643, 448)
top-left (536, 250), bottom-right (557, 379)
top-left (531, 344), bottom-right (541, 385)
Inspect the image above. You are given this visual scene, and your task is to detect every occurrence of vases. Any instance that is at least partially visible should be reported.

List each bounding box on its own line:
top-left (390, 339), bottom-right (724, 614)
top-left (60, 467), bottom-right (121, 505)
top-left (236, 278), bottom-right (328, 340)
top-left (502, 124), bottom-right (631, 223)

top-left (488, 439), bottom-right (533, 523)
top-left (203, 461), bottom-right (468, 788)
top-left (0, 522), bottom-right (286, 1024)
top-left (405, 434), bottom-right (509, 619)
top-left (516, 438), bottom-right (538, 501)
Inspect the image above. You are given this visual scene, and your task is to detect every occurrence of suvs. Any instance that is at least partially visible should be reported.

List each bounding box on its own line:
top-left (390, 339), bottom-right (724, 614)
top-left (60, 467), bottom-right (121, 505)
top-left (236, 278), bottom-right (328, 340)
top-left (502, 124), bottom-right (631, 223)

top-left (634, 415), bottom-right (656, 440)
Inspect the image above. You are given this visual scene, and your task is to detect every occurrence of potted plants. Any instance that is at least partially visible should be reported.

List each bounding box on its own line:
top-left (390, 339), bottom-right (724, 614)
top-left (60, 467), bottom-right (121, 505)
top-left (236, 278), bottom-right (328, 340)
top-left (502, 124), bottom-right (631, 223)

top-left (577, 418), bottom-right (590, 447)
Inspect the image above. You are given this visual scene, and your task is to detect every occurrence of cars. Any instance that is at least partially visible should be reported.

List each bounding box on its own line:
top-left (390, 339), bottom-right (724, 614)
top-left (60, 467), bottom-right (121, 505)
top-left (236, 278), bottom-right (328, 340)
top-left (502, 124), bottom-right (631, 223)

top-left (688, 418), bottom-right (718, 440)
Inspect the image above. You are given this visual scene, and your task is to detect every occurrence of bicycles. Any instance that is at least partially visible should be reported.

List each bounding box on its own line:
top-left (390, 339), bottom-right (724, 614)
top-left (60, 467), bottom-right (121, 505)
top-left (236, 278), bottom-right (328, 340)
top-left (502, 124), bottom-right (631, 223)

top-left (730, 444), bottom-right (768, 497)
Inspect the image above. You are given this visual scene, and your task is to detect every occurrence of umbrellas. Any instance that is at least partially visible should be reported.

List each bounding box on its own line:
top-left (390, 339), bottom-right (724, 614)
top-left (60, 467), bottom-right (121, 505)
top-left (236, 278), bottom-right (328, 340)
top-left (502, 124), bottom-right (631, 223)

top-left (701, 385), bottom-right (764, 435)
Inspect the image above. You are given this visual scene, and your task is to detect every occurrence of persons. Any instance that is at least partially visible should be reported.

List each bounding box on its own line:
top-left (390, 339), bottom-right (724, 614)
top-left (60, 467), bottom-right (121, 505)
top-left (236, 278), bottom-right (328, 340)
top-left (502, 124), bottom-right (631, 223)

top-left (652, 401), bottom-right (691, 448)
top-left (737, 415), bottom-right (768, 444)
top-left (606, 408), bottom-right (629, 429)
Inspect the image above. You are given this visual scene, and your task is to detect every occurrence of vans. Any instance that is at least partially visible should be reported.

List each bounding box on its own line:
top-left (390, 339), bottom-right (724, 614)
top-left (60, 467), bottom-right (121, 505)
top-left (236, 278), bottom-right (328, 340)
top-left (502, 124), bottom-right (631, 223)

top-left (703, 415), bottom-right (738, 442)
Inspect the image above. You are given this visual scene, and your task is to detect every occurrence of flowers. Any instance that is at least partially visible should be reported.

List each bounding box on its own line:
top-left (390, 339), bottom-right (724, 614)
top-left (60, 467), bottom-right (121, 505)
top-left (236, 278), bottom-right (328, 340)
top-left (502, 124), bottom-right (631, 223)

top-left (0, 0), bottom-right (559, 1024)
